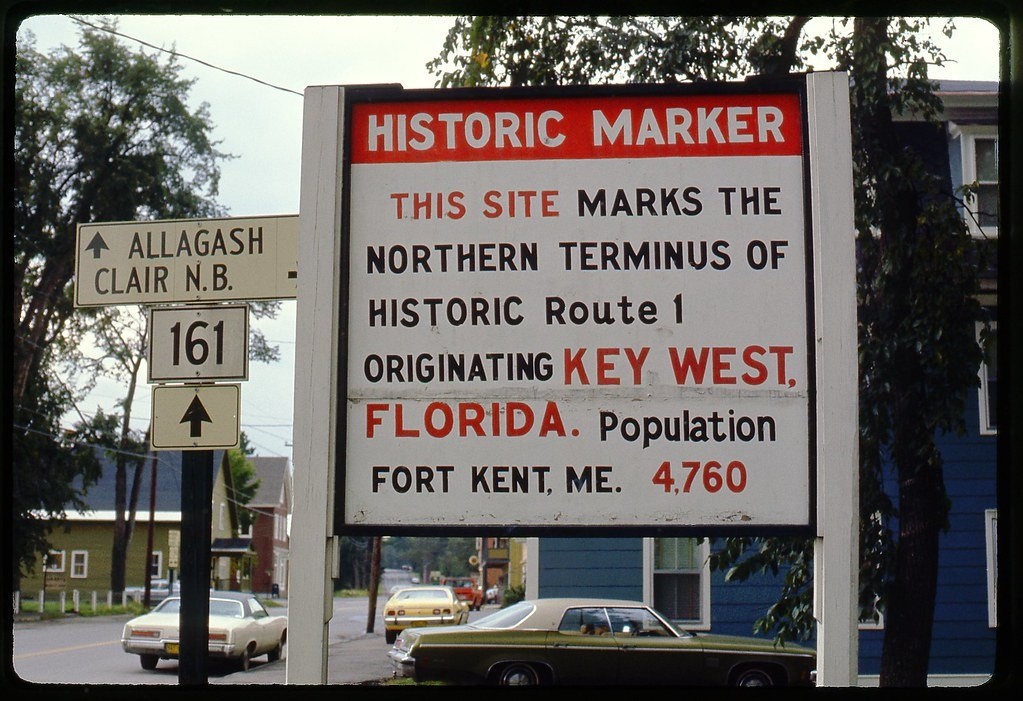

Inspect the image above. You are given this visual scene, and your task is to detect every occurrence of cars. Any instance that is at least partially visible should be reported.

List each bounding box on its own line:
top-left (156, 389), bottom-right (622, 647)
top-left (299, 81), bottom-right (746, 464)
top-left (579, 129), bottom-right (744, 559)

top-left (383, 577), bottom-right (483, 643)
top-left (121, 591), bottom-right (289, 673)
top-left (385, 597), bottom-right (816, 689)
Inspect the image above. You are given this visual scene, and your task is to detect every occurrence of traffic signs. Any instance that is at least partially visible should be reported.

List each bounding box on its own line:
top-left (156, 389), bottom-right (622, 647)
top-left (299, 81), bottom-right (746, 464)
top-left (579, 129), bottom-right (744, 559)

top-left (73, 214), bottom-right (302, 307)
